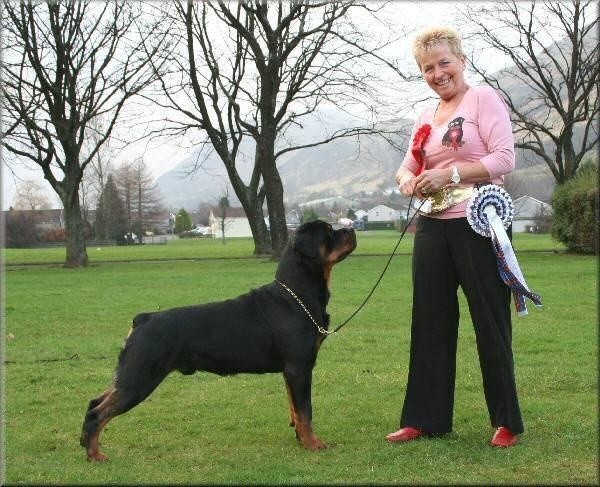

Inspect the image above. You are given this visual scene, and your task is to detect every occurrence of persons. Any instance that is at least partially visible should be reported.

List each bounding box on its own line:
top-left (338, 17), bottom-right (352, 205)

top-left (384, 28), bottom-right (543, 448)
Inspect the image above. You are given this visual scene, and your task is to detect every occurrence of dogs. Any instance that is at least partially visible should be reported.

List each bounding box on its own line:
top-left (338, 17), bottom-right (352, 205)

top-left (79, 220), bottom-right (357, 464)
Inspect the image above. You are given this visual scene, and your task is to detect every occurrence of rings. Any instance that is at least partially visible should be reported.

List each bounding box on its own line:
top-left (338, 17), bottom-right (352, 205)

top-left (421, 186), bottom-right (427, 192)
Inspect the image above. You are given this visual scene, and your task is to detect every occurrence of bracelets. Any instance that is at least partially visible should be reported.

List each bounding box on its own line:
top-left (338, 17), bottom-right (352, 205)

top-left (452, 165), bottom-right (461, 183)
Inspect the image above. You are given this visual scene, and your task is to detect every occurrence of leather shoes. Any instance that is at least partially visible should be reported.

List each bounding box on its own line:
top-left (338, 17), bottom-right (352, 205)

top-left (491, 426), bottom-right (520, 447)
top-left (386, 426), bottom-right (425, 442)
